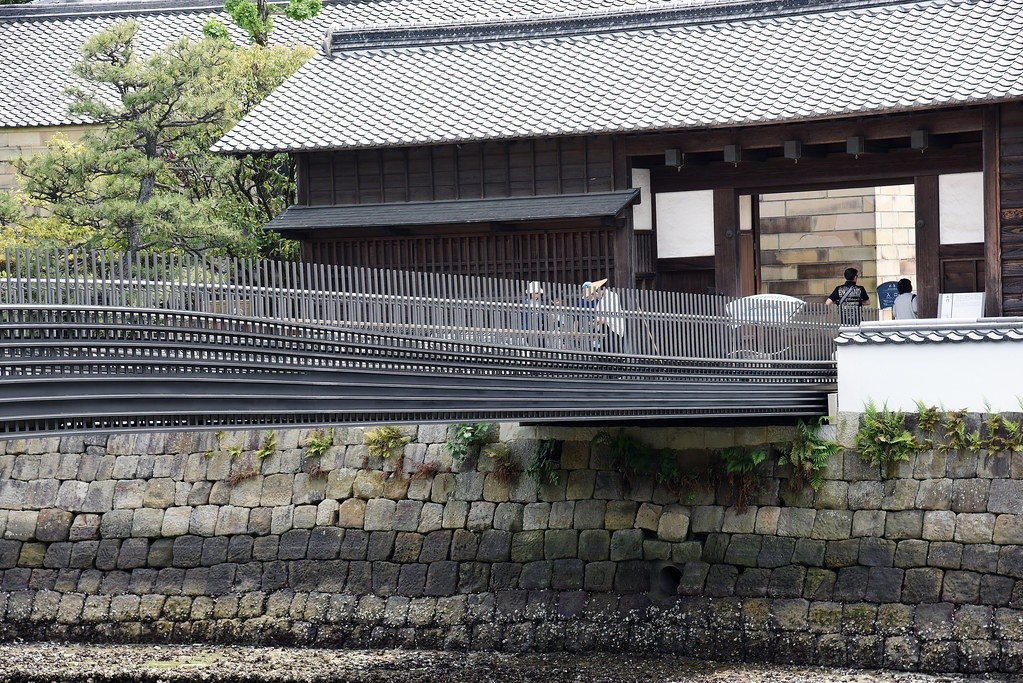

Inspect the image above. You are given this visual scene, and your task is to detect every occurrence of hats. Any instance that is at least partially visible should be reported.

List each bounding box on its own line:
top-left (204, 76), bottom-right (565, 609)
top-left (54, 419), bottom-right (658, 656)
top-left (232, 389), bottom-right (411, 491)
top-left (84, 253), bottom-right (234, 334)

top-left (526, 280), bottom-right (544, 294)
top-left (582, 282), bottom-right (592, 290)
top-left (582, 278), bottom-right (608, 299)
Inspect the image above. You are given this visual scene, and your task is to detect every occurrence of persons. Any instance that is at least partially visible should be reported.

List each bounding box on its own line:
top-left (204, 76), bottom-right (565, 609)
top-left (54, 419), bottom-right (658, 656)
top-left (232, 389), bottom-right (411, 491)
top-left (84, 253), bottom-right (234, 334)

top-left (892, 278), bottom-right (917, 320)
top-left (577, 278), bottom-right (624, 353)
top-left (826, 268), bottom-right (870, 325)
top-left (522, 281), bottom-right (564, 348)
